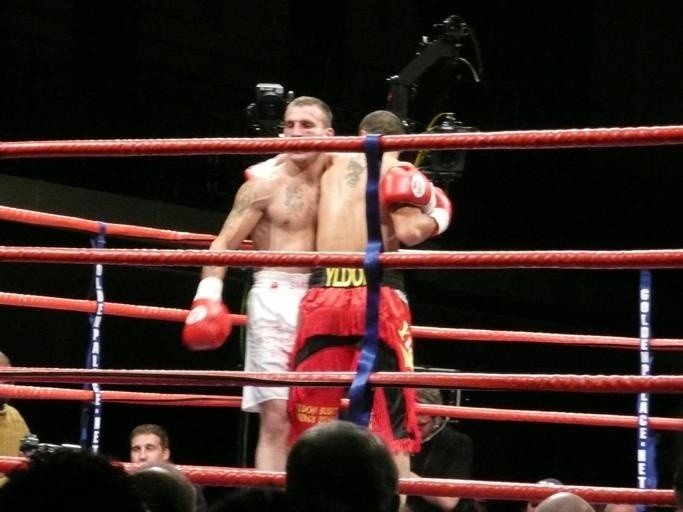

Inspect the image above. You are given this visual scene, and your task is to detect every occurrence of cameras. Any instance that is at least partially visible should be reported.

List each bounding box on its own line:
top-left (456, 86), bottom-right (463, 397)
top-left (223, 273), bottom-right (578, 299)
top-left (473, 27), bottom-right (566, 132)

top-left (245, 82), bottom-right (293, 122)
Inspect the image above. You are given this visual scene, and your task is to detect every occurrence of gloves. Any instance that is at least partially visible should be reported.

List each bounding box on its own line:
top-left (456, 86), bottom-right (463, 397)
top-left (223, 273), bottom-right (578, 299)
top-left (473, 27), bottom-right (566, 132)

top-left (380, 167), bottom-right (436, 212)
top-left (182, 277), bottom-right (232, 350)
top-left (428, 185), bottom-right (452, 237)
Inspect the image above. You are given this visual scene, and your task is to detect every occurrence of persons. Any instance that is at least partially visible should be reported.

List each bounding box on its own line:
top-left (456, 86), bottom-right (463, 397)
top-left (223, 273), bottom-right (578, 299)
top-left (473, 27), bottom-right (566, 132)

top-left (178, 95), bottom-right (435, 474)
top-left (0, 418), bottom-right (683, 511)
top-left (408, 366), bottom-right (477, 511)
top-left (240, 106), bottom-right (456, 511)
top-left (0, 349), bottom-right (31, 487)
